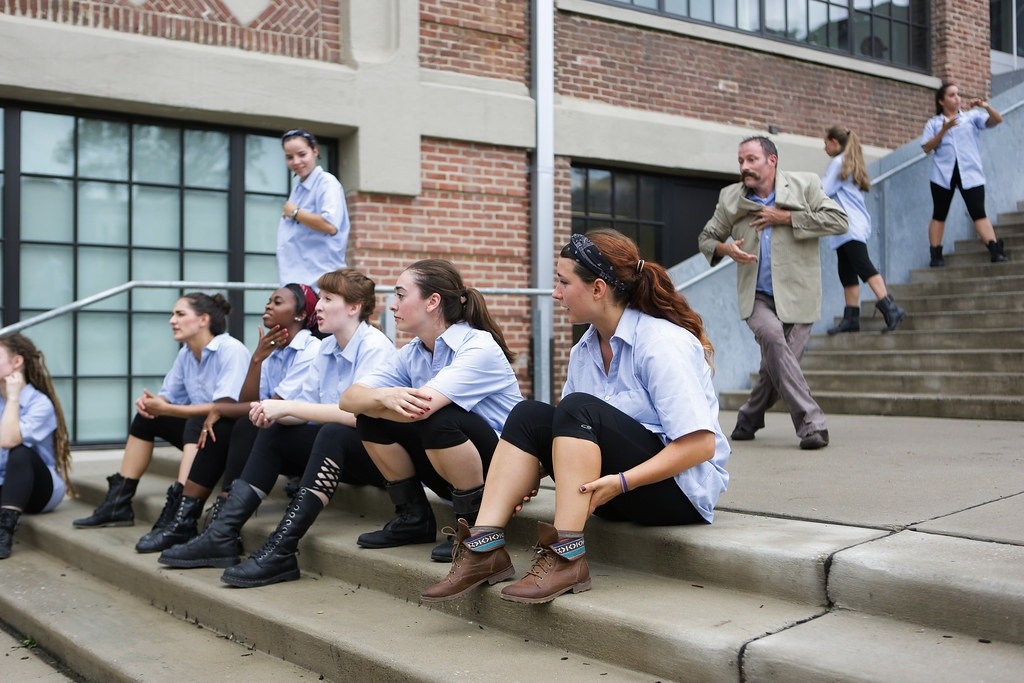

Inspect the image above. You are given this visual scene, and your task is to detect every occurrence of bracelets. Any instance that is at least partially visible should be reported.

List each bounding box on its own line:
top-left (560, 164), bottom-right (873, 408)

top-left (619, 472), bottom-right (629, 493)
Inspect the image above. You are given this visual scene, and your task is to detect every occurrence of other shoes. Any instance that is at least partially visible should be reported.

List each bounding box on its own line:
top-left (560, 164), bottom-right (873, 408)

top-left (797, 431), bottom-right (829, 449)
top-left (732, 423), bottom-right (756, 442)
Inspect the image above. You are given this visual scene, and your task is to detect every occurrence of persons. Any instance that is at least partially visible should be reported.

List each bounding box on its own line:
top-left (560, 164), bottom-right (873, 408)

top-left (73, 292), bottom-right (251, 539)
top-left (422, 230), bottom-right (732, 603)
top-left (698, 137), bottom-right (848, 448)
top-left (823, 124), bottom-right (908, 334)
top-left (276, 129), bottom-right (350, 294)
top-left (920, 83), bottom-right (1008, 267)
top-left (135, 283), bottom-right (334, 553)
top-left (340, 259), bottom-right (525, 563)
top-left (0, 330), bottom-right (72, 559)
top-left (157, 267), bottom-right (398, 588)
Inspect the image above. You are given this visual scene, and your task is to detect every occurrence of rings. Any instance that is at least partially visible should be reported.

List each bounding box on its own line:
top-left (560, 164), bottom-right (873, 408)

top-left (270, 341), bottom-right (275, 345)
top-left (203, 430), bottom-right (207, 432)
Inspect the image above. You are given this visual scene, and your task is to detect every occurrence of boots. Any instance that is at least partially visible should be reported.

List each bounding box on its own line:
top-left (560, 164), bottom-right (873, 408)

top-left (501, 520), bottom-right (592, 604)
top-left (136, 498), bottom-right (208, 553)
top-left (0, 509), bottom-right (21, 559)
top-left (986, 239), bottom-right (1008, 262)
top-left (877, 294), bottom-right (905, 333)
top-left (431, 486), bottom-right (487, 562)
top-left (151, 482), bottom-right (184, 531)
top-left (157, 478), bottom-right (262, 568)
top-left (357, 477), bottom-right (439, 549)
top-left (205, 495), bottom-right (247, 555)
top-left (421, 524), bottom-right (515, 603)
top-left (223, 486), bottom-right (326, 587)
top-left (72, 474), bottom-right (136, 529)
top-left (928, 243), bottom-right (943, 266)
top-left (826, 306), bottom-right (860, 336)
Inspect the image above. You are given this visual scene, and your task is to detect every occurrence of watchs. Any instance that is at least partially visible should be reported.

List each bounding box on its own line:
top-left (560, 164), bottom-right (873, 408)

top-left (291, 208), bottom-right (300, 221)
